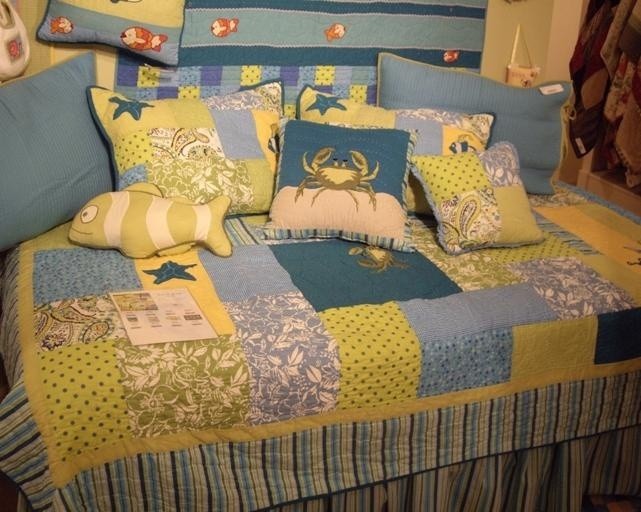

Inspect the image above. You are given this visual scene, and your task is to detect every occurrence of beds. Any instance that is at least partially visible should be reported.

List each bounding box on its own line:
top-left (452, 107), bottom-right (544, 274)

top-left (1, 2), bottom-right (640, 512)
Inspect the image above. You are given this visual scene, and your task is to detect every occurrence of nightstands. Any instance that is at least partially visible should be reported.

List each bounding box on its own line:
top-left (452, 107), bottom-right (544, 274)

top-left (588, 170), bottom-right (641, 220)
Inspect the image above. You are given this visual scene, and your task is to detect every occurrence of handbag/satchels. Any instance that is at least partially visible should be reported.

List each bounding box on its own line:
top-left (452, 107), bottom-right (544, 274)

top-left (506, 26), bottom-right (540, 91)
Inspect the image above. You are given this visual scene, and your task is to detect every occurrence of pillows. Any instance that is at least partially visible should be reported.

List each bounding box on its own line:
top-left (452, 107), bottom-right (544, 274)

top-left (407, 139), bottom-right (547, 252)
top-left (37, 0), bottom-right (188, 67)
top-left (88, 78), bottom-right (286, 222)
top-left (264, 114), bottom-right (420, 258)
top-left (376, 52), bottom-right (572, 199)
top-left (0, 51), bottom-right (115, 258)
top-left (294, 81), bottom-right (498, 223)
